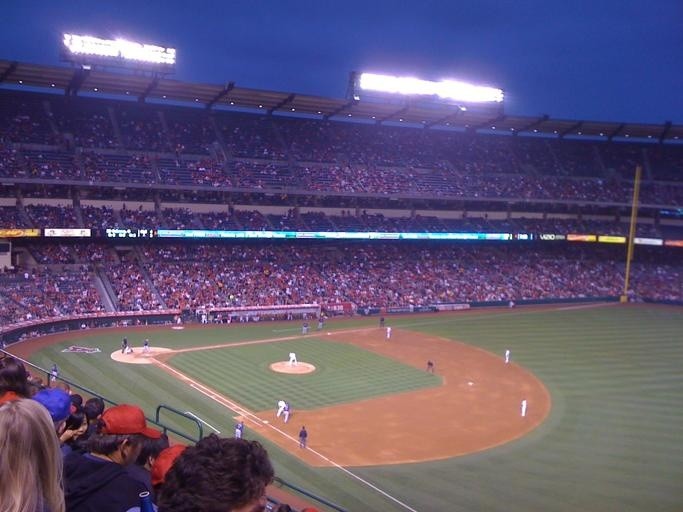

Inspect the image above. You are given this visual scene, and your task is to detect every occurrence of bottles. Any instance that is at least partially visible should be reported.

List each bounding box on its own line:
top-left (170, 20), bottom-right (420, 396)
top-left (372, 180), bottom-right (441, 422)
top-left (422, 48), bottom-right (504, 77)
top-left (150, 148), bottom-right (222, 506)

top-left (138, 492), bottom-right (154, 512)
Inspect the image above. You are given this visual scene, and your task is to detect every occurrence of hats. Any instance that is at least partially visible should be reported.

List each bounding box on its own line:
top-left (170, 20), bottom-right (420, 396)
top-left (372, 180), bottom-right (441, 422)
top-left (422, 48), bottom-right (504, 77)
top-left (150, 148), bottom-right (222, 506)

top-left (95, 404), bottom-right (161, 439)
top-left (31, 387), bottom-right (77, 422)
top-left (151, 444), bottom-right (187, 493)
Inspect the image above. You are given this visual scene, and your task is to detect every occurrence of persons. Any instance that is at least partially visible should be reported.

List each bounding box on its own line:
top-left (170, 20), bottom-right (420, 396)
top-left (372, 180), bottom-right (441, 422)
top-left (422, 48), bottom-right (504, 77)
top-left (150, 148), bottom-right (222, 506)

top-left (303, 318), bottom-right (324, 334)
top-left (521, 399), bottom-right (526, 416)
top-left (2, 96), bottom-right (682, 324)
top-left (505, 349), bottom-right (510, 364)
top-left (277, 399), bottom-right (307, 449)
top-left (380, 316), bottom-right (392, 339)
top-left (121, 336), bottom-right (150, 354)
top-left (289, 352), bottom-right (297, 367)
top-left (0, 357), bottom-right (274, 512)
top-left (427, 360), bottom-right (433, 374)
